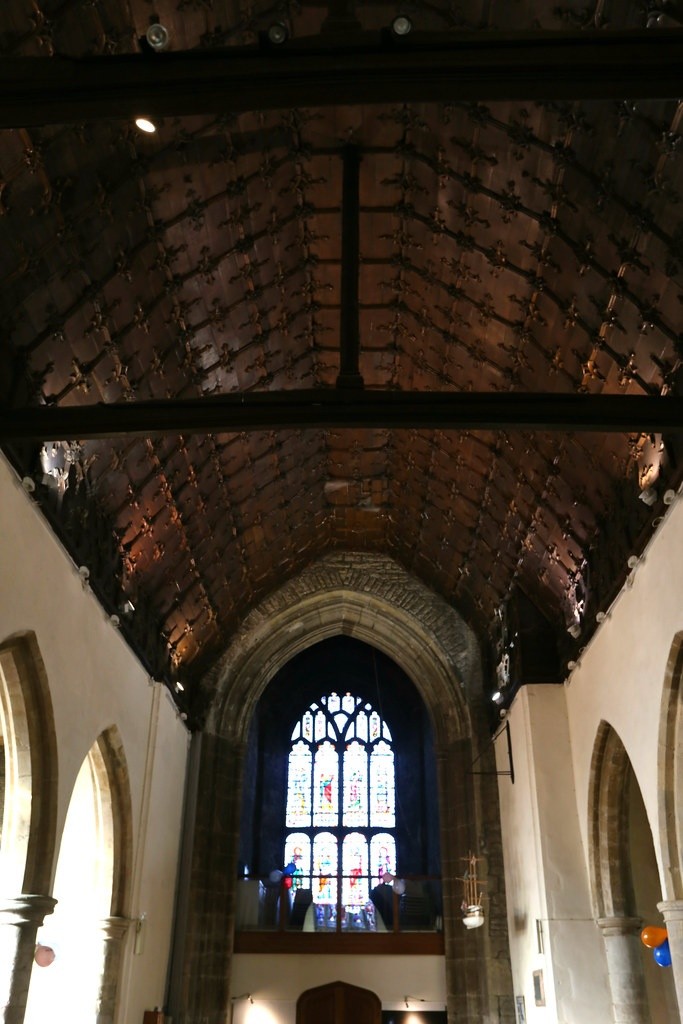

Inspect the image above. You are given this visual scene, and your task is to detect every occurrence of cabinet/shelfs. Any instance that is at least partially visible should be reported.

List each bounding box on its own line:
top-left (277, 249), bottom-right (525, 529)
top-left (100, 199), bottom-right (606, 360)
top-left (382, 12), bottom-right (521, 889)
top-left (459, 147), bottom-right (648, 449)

top-left (235, 880), bottom-right (268, 929)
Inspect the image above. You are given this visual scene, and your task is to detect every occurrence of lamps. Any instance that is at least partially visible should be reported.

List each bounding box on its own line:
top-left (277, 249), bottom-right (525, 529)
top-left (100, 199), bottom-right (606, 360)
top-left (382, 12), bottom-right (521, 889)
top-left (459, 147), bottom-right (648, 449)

top-left (176, 711), bottom-right (188, 720)
top-left (21, 476), bottom-right (36, 492)
top-left (627, 556), bottom-right (641, 569)
top-left (566, 661), bottom-right (578, 671)
top-left (78, 565), bottom-right (90, 581)
top-left (596, 611), bottom-right (607, 624)
top-left (135, 116), bottom-right (164, 134)
top-left (110, 614), bottom-right (121, 627)
top-left (174, 677), bottom-right (188, 693)
top-left (663, 490), bottom-right (680, 505)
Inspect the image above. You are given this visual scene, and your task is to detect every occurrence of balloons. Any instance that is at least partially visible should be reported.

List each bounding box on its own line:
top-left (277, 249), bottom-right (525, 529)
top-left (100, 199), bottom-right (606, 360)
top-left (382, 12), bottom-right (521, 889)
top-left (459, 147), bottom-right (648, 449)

top-left (269, 863), bottom-right (296, 889)
top-left (383, 872), bottom-right (393, 883)
top-left (35, 946), bottom-right (55, 967)
top-left (641, 926), bottom-right (672, 968)
top-left (392, 879), bottom-right (406, 894)
